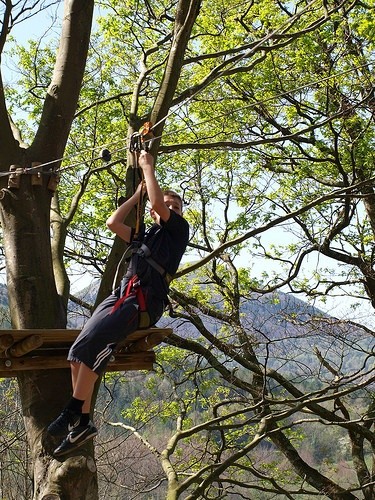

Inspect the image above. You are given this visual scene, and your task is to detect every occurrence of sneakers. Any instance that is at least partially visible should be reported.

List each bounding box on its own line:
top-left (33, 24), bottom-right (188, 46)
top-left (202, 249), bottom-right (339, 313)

top-left (47, 413), bottom-right (82, 438)
top-left (53, 420), bottom-right (98, 456)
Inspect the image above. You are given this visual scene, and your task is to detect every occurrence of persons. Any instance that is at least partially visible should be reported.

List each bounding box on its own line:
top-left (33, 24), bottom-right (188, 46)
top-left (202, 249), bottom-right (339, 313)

top-left (45, 151), bottom-right (190, 456)
